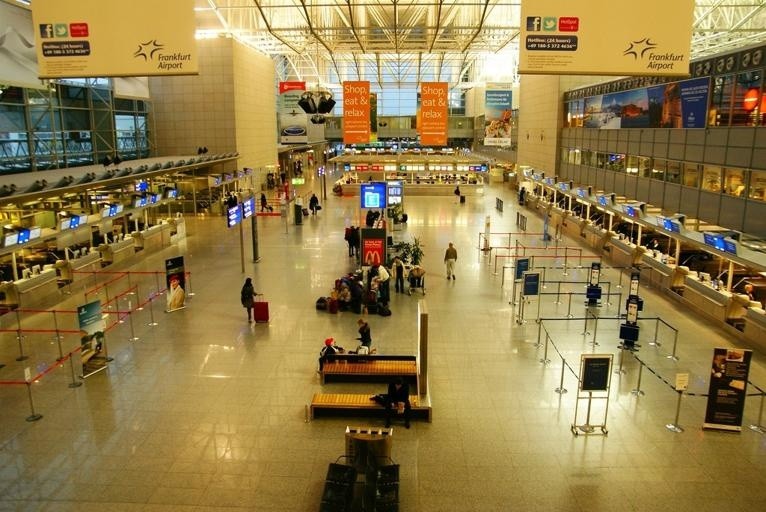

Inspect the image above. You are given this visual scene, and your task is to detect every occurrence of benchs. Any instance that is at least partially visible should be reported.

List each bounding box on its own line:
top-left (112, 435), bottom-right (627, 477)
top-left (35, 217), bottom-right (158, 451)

top-left (308, 359), bottom-right (433, 422)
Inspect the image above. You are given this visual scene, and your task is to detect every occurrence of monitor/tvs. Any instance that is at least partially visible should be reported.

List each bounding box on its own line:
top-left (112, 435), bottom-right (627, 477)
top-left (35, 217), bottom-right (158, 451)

top-left (1, 167), bottom-right (254, 306)
top-left (523, 168), bottom-right (742, 283)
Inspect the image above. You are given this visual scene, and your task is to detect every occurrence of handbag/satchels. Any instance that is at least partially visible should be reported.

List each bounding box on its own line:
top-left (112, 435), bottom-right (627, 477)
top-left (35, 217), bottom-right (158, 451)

top-left (411, 269), bottom-right (425, 277)
top-left (316, 206), bottom-right (321, 210)
top-left (316, 296), bottom-right (346, 314)
top-left (378, 308), bottom-right (391, 316)
top-left (368, 291), bottom-right (379, 314)
top-left (345, 229), bottom-right (351, 240)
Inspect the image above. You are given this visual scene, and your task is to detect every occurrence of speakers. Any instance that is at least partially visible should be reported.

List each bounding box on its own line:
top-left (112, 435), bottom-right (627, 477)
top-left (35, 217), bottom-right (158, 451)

top-left (298, 98), bottom-right (317, 114)
top-left (318, 97), bottom-right (336, 114)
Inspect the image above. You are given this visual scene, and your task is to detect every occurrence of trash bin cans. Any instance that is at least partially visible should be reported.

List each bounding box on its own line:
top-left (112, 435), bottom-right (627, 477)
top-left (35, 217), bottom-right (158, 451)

top-left (461, 196), bottom-right (465, 202)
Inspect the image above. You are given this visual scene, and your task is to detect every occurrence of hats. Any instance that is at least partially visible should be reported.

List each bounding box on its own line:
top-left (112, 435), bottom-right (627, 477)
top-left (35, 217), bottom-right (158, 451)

top-left (325, 339), bottom-right (333, 346)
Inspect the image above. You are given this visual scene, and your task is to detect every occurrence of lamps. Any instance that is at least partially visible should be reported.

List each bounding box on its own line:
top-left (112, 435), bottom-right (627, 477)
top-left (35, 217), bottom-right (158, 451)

top-left (296, 0), bottom-right (337, 129)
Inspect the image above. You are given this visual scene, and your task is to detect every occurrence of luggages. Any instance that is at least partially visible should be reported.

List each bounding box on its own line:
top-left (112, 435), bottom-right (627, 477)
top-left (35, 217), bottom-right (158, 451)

top-left (253, 294), bottom-right (268, 322)
top-left (303, 209), bottom-right (309, 216)
top-left (357, 346), bottom-right (368, 362)
top-left (401, 215), bottom-right (407, 223)
top-left (460, 196), bottom-right (465, 203)
top-left (410, 277), bottom-right (420, 287)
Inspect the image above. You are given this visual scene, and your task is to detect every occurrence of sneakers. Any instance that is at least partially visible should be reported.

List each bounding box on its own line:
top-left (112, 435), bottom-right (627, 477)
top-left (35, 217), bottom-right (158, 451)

top-left (447, 275), bottom-right (456, 280)
top-left (405, 420), bottom-right (410, 428)
top-left (384, 422), bottom-right (391, 428)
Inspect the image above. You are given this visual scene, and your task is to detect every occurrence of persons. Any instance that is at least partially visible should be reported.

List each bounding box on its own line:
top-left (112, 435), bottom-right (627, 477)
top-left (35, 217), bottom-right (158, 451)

top-left (166, 273), bottom-right (185, 313)
top-left (391, 256), bottom-right (407, 294)
top-left (239, 277), bottom-right (257, 325)
top-left (453, 185), bottom-right (459, 205)
top-left (442, 242), bottom-right (456, 281)
top-left (744, 284), bottom-right (756, 301)
top-left (309, 193), bottom-right (318, 216)
top-left (372, 263), bottom-right (391, 308)
top-left (227, 191), bottom-right (236, 207)
top-left (317, 337), bottom-right (344, 369)
top-left (652, 239), bottom-right (664, 254)
top-left (519, 187), bottom-right (525, 205)
top-left (384, 377), bottom-right (411, 431)
top-left (354, 319), bottom-right (372, 353)
top-left (353, 226), bottom-right (361, 259)
top-left (346, 225), bottom-right (355, 258)
top-left (259, 192), bottom-right (268, 212)
